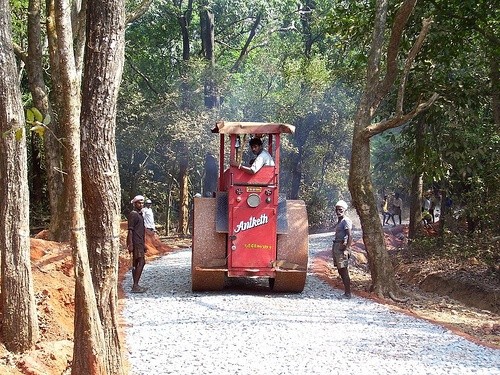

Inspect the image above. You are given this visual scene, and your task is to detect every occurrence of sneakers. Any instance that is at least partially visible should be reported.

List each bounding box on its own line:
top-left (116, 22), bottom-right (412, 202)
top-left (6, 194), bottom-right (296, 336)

top-left (131, 285), bottom-right (148, 293)
top-left (338, 294), bottom-right (352, 299)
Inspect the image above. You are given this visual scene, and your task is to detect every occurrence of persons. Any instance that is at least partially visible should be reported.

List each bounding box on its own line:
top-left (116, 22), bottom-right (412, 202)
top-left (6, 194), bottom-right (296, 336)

top-left (128, 195), bottom-right (148, 292)
top-left (332, 200), bottom-right (352, 299)
top-left (445, 198), bottom-right (452, 215)
top-left (238, 137), bottom-right (275, 174)
top-left (422, 194), bottom-right (435, 224)
top-left (390, 192), bottom-right (402, 227)
top-left (381, 195), bottom-right (392, 226)
top-left (141, 199), bottom-right (155, 234)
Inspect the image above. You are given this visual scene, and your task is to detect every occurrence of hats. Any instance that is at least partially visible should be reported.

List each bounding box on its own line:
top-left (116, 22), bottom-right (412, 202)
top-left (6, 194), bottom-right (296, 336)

top-left (131, 195), bottom-right (144, 204)
top-left (336, 200), bottom-right (347, 210)
top-left (145, 199), bottom-right (152, 204)
top-left (193, 193), bottom-right (202, 198)
top-left (204, 192), bottom-right (213, 198)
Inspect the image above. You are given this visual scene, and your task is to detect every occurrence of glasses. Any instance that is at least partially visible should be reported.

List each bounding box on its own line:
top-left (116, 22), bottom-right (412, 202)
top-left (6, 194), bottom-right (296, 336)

top-left (335, 209), bottom-right (344, 211)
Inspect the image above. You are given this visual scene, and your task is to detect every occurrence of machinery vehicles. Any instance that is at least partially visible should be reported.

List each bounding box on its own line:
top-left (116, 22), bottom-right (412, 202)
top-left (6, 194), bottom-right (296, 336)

top-left (190, 119), bottom-right (310, 294)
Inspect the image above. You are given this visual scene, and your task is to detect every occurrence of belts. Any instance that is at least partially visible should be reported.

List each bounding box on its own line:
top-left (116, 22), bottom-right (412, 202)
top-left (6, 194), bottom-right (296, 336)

top-left (333, 240), bottom-right (345, 243)
